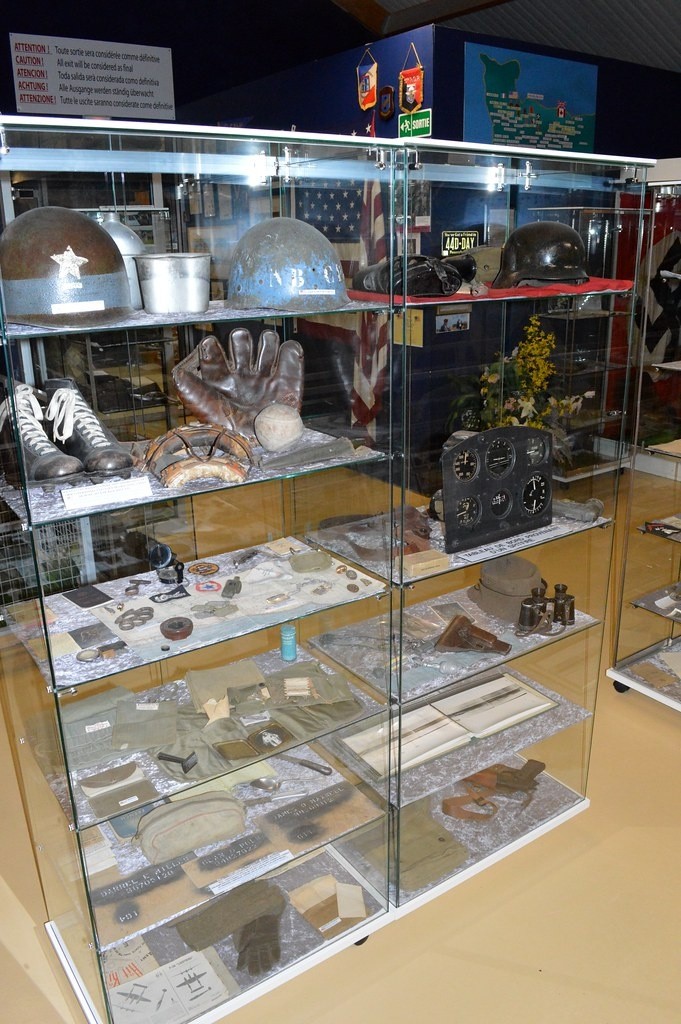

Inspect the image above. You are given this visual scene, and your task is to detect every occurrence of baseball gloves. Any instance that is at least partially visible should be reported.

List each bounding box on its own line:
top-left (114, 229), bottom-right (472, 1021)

top-left (169, 327), bottom-right (307, 449)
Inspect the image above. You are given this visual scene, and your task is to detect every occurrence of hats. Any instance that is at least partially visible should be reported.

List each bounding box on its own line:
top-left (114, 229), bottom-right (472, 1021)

top-left (466, 554), bottom-right (547, 620)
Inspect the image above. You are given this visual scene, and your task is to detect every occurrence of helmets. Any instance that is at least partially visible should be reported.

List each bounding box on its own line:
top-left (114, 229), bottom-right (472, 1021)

top-left (226, 217), bottom-right (353, 312)
top-left (489, 222), bottom-right (590, 290)
top-left (1, 205), bottom-right (133, 328)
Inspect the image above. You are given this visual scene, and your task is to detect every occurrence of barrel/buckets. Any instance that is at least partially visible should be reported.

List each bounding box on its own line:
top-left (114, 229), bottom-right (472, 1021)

top-left (132, 253), bottom-right (212, 316)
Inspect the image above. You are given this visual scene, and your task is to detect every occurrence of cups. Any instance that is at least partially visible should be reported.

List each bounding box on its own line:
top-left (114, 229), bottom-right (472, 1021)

top-left (280, 625), bottom-right (296, 662)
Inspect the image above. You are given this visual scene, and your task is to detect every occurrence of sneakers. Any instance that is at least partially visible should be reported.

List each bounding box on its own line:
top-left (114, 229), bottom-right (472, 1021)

top-left (0, 384), bottom-right (85, 486)
top-left (41, 377), bottom-right (134, 478)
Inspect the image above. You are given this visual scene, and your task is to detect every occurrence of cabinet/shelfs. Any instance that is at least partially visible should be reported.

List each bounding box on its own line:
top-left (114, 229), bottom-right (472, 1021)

top-left (0, 114), bottom-right (681, 1024)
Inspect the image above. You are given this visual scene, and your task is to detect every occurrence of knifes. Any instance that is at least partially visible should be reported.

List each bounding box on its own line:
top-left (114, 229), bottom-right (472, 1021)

top-left (276, 754), bottom-right (332, 776)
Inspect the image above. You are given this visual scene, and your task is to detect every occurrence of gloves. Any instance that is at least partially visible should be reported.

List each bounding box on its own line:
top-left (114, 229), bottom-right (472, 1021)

top-left (171, 327), bottom-right (304, 448)
top-left (176, 881), bottom-right (285, 975)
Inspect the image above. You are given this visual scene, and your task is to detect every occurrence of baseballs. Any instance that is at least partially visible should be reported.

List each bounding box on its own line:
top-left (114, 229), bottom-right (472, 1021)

top-left (254, 402), bottom-right (304, 454)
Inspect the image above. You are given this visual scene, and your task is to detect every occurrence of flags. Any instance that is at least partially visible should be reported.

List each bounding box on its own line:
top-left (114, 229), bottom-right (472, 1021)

top-left (295, 179), bottom-right (366, 244)
top-left (351, 108), bottom-right (389, 442)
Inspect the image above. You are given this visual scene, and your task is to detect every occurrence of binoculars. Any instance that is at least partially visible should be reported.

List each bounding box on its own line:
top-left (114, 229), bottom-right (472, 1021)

top-left (518, 583), bottom-right (577, 631)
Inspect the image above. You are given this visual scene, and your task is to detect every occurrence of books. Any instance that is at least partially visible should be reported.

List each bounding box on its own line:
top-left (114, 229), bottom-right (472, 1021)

top-left (61, 583), bottom-right (114, 609)
top-left (288, 874), bottom-right (373, 940)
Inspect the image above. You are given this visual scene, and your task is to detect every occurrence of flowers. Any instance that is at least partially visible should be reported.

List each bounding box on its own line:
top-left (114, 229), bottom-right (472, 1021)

top-left (445, 311), bottom-right (596, 468)
